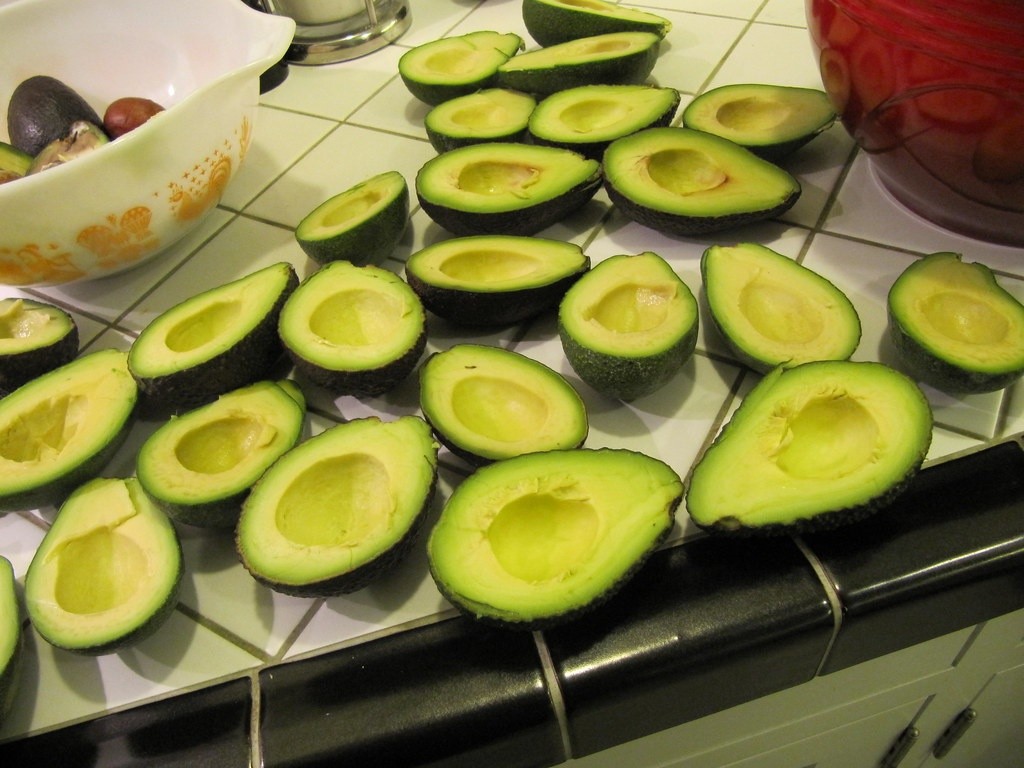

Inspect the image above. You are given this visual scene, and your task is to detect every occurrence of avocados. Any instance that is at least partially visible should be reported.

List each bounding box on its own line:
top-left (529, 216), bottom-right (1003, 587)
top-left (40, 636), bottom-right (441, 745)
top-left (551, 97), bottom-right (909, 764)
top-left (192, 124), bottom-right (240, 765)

top-left (0, 74), bottom-right (112, 185)
top-left (0, 172), bottom-right (439, 728)
top-left (399, 0), bottom-right (1024, 634)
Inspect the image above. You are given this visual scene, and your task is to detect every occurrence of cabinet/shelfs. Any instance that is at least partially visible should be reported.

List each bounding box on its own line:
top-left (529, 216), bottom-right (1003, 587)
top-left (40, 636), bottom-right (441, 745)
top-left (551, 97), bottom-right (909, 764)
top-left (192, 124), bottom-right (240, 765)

top-left (552, 607), bottom-right (1024, 768)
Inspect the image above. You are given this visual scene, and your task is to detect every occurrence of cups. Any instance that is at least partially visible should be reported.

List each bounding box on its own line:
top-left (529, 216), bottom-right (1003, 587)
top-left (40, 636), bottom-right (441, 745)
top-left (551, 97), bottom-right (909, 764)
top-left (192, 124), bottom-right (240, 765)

top-left (254, 1), bottom-right (412, 66)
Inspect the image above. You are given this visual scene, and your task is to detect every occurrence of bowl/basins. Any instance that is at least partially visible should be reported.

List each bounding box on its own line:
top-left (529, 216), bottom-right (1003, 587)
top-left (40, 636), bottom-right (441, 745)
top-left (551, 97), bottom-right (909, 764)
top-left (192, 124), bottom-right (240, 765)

top-left (804, 0), bottom-right (1023, 251)
top-left (1, 1), bottom-right (296, 283)
